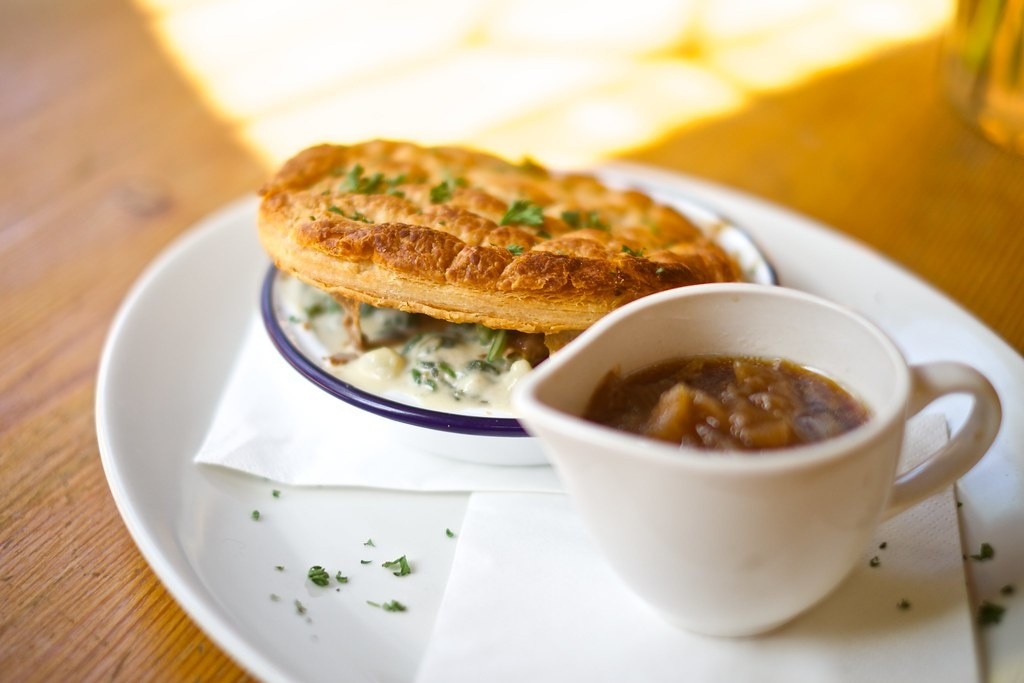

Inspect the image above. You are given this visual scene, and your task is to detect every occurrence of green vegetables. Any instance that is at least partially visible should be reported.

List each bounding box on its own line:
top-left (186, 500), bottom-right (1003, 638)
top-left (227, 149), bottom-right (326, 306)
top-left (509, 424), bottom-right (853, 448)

top-left (250, 486), bottom-right (455, 613)
top-left (288, 165), bottom-right (669, 400)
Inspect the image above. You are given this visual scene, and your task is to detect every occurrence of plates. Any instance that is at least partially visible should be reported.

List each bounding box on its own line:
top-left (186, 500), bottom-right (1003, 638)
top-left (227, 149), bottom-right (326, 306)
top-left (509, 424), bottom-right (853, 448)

top-left (95, 156), bottom-right (1024, 683)
top-left (258, 187), bottom-right (782, 466)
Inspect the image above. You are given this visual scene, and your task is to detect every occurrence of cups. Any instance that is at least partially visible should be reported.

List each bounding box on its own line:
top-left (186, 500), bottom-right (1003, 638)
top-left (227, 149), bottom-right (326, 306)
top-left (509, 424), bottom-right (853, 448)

top-left (509, 282), bottom-right (1004, 639)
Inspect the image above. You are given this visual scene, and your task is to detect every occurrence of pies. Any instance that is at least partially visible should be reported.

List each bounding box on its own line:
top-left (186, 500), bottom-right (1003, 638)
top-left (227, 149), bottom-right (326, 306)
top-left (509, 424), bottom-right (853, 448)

top-left (257, 139), bottom-right (740, 328)
